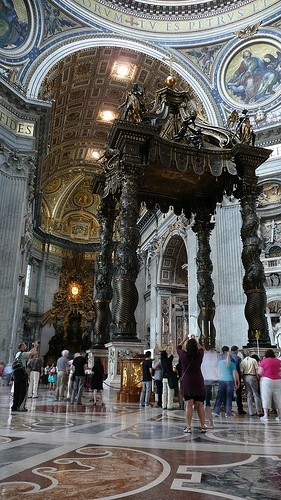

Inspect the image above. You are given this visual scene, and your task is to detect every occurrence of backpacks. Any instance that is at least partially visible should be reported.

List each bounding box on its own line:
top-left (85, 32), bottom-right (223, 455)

top-left (11, 351), bottom-right (28, 383)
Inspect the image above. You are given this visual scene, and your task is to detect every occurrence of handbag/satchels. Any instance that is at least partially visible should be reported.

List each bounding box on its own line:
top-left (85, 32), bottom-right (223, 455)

top-left (178, 377), bottom-right (182, 392)
top-left (167, 370), bottom-right (179, 389)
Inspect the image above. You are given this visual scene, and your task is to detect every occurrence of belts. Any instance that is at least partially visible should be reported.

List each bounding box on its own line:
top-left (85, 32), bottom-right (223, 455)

top-left (244, 374), bottom-right (257, 376)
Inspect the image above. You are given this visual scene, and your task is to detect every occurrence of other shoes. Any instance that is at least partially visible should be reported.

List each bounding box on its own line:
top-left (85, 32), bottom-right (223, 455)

top-left (93, 403), bottom-right (96, 405)
top-left (77, 403), bottom-right (82, 405)
top-left (102, 403), bottom-right (105, 406)
top-left (11, 406), bottom-right (28, 411)
top-left (28, 396), bottom-right (32, 398)
top-left (33, 396), bottom-right (38, 399)
top-left (239, 410), bottom-right (247, 414)
top-left (225, 413), bottom-right (235, 418)
top-left (211, 412), bottom-right (219, 417)
top-left (270, 410), bottom-right (277, 414)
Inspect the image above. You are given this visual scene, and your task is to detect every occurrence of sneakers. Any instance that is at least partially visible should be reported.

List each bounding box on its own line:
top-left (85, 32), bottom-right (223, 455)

top-left (182, 427), bottom-right (192, 432)
top-left (145, 404), bottom-right (152, 408)
top-left (199, 428), bottom-right (206, 433)
top-left (275, 416), bottom-right (281, 421)
top-left (260, 416), bottom-right (269, 421)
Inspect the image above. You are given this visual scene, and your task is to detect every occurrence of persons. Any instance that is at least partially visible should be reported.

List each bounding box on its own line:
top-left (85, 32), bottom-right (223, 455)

top-left (11, 341), bottom-right (59, 411)
top-left (176, 334), bottom-right (205, 433)
top-left (0, 362), bottom-right (4, 386)
top-left (239, 110), bottom-right (255, 143)
top-left (116, 82), bottom-right (150, 125)
top-left (138, 341), bottom-right (281, 431)
top-left (174, 109), bottom-right (205, 148)
top-left (56, 350), bottom-right (69, 401)
top-left (63, 350), bottom-right (88, 405)
top-left (92, 358), bottom-right (104, 406)
